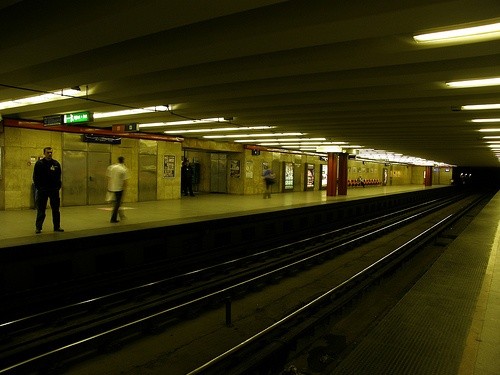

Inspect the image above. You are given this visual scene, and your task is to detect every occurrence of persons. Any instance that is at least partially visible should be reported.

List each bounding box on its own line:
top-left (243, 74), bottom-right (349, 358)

top-left (261, 164), bottom-right (276, 199)
top-left (181, 160), bottom-right (194, 197)
top-left (104, 156), bottom-right (130, 223)
top-left (32, 147), bottom-right (65, 233)
top-left (357, 175), bottom-right (365, 188)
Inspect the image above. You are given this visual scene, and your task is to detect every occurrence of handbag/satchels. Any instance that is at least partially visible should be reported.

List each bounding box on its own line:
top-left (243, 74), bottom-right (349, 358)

top-left (106, 192), bottom-right (114, 202)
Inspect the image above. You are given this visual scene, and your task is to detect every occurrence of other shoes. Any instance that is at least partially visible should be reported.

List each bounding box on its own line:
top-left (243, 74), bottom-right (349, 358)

top-left (190, 194), bottom-right (195, 197)
top-left (35, 229), bottom-right (40, 233)
top-left (54, 228), bottom-right (64, 232)
top-left (111, 219), bottom-right (120, 223)
top-left (185, 193), bottom-right (188, 195)
